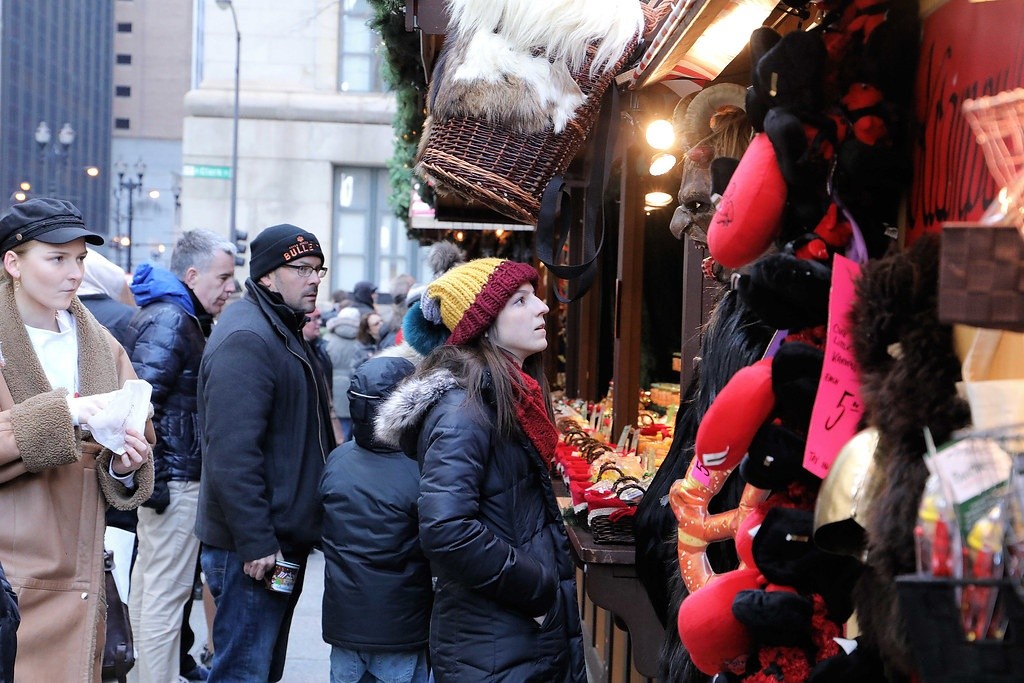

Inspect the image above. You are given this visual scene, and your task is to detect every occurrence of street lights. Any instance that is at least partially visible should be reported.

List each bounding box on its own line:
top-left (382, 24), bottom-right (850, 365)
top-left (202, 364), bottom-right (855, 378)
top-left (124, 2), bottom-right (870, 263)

top-left (215, 0), bottom-right (240, 280)
top-left (34, 119), bottom-right (76, 201)
top-left (113, 152), bottom-right (148, 271)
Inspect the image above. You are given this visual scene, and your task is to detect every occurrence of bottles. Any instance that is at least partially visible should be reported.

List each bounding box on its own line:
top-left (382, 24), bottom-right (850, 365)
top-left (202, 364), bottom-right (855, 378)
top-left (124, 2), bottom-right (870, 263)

top-left (650, 382), bottom-right (680, 410)
top-left (917, 470), bottom-right (1023, 639)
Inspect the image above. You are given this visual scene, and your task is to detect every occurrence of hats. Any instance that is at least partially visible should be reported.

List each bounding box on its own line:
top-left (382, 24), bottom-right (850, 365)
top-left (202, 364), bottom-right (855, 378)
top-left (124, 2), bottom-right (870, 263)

top-left (392, 274), bottom-right (417, 302)
top-left (403, 258), bottom-right (539, 356)
top-left (249, 225), bottom-right (324, 281)
top-left (1, 198), bottom-right (104, 253)
top-left (339, 307), bottom-right (361, 321)
top-left (405, 283), bottom-right (426, 306)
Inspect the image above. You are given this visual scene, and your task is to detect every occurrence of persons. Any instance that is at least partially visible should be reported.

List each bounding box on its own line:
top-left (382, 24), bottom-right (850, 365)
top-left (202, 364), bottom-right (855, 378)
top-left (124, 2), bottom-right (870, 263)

top-left (318, 355), bottom-right (429, 683)
top-left (193, 225), bottom-right (340, 682)
top-left (76, 244), bottom-right (415, 683)
top-left (1, 198), bottom-right (158, 682)
top-left (373, 256), bottom-right (590, 682)
top-left (118, 231), bottom-right (236, 683)
top-left (633, 286), bottom-right (778, 683)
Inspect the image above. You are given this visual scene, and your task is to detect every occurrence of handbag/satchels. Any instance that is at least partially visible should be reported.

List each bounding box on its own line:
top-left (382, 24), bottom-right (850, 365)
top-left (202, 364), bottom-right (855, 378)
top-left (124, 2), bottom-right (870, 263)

top-left (98, 550), bottom-right (135, 681)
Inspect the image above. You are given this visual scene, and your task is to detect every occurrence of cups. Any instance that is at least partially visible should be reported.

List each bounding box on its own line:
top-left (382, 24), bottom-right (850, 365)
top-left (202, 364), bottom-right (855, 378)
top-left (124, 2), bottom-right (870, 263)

top-left (265, 559), bottom-right (300, 594)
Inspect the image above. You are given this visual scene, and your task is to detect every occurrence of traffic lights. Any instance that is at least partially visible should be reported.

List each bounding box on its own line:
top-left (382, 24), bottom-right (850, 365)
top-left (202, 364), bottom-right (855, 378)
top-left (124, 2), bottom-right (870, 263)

top-left (234, 230), bottom-right (250, 269)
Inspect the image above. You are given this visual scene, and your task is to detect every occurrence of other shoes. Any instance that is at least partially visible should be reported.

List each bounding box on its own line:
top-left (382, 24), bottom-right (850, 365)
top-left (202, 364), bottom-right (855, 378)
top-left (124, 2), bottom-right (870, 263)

top-left (182, 664), bottom-right (209, 680)
top-left (200, 645), bottom-right (214, 668)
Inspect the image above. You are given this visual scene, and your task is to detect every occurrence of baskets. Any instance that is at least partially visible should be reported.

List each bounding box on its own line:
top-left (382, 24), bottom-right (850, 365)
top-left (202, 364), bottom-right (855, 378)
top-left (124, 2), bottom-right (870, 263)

top-left (549, 419), bottom-right (648, 544)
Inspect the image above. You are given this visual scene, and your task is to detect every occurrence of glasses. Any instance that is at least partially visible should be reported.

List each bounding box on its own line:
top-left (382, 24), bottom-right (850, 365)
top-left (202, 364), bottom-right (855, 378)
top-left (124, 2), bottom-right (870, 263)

top-left (284, 264), bottom-right (329, 278)
